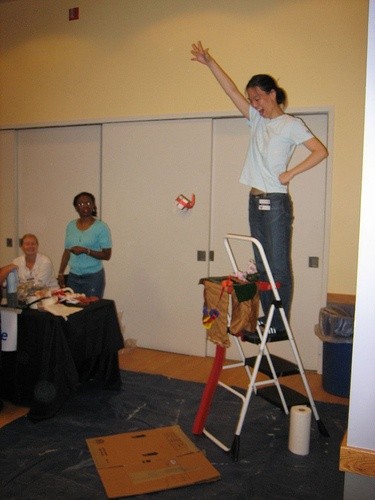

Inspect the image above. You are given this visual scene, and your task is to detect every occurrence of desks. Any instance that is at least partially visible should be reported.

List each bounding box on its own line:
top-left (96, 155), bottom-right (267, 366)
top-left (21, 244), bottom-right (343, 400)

top-left (0, 297), bottom-right (127, 413)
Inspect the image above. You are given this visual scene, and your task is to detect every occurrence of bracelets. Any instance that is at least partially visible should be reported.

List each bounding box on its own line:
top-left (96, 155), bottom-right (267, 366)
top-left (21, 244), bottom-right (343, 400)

top-left (58, 272), bottom-right (64, 276)
top-left (86, 247), bottom-right (92, 255)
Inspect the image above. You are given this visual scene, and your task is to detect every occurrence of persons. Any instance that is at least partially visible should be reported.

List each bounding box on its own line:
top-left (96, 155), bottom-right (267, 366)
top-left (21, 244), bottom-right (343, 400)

top-left (189, 39), bottom-right (330, 336)
top-left (56, 192), bottom-right (111, 299)
top-left (0, 262), bottom-right (20, 284)
top-left (11, 233), bottom-right (59, 290)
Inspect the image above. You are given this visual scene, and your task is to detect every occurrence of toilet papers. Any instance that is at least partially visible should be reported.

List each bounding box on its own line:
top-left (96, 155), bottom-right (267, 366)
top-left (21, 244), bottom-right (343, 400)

top-left (288, 405), bottom-right (312, 455)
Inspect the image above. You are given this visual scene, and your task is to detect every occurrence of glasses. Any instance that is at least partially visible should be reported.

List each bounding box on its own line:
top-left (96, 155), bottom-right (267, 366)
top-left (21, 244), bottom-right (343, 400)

top-left (76, 202), bottom-right (93, 207)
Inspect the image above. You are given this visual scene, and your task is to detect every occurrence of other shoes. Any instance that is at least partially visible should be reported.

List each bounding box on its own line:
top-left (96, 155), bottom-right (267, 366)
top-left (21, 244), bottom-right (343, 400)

top-left (257, 318), bottom-right (286, 335)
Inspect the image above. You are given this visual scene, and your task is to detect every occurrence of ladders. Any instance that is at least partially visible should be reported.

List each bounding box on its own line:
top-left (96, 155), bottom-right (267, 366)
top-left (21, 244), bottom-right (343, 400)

top-left (191, 233), bottom-right (331, 464)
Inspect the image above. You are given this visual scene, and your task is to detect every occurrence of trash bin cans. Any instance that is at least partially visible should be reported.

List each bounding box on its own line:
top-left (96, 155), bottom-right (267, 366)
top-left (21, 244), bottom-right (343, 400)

top-left (313, 302), bottom-right (355, 398)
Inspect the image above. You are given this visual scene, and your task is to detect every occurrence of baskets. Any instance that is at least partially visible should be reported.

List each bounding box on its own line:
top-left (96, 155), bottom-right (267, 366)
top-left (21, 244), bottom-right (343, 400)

top-left (203, 276), bottom-right (259, 328)
